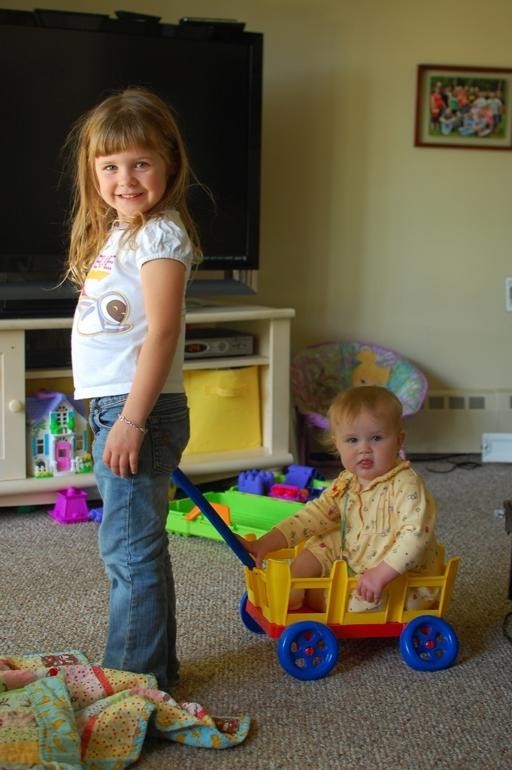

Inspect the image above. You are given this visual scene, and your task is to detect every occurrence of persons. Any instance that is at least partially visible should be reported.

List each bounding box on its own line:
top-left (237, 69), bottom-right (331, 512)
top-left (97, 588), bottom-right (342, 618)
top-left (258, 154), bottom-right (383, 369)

top-left (227, 384), bottom-right (446, 610)
top-left (426, 76), bottom-right (509, 139)
top-left (38, 85), bottom-right (206, 686)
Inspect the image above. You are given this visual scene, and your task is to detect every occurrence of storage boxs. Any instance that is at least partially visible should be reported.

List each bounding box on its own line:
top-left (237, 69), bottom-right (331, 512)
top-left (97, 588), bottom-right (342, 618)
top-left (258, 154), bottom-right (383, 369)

top-left (179, 365), bottom-right (262, 456)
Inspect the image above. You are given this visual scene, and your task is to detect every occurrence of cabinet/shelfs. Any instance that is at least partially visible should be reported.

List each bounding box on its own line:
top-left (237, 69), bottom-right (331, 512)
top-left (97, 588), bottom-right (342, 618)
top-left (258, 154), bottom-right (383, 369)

top-left (0, 302), bottom-right (295, 508)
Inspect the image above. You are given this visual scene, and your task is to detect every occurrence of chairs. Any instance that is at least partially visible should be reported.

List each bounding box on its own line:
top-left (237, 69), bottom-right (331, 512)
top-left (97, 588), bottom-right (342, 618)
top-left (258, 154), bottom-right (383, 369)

top-left (289, 339), bottom-right (429, 468)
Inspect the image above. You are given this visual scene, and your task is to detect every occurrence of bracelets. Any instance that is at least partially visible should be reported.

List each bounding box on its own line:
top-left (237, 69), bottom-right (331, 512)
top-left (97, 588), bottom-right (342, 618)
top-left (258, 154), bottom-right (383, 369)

top-left (117, 410), bottom-right (149, 437)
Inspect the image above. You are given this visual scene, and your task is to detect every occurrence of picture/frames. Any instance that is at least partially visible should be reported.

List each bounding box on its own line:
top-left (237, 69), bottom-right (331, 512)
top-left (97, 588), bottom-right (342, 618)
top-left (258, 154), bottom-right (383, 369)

top-left (414, 64), bottom-right (512, 151)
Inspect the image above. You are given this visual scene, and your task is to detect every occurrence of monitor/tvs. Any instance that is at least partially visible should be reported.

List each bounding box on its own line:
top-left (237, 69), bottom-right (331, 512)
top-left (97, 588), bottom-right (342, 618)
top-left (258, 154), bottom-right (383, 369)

top-left (1, 8), bottom-right (263, 318)
